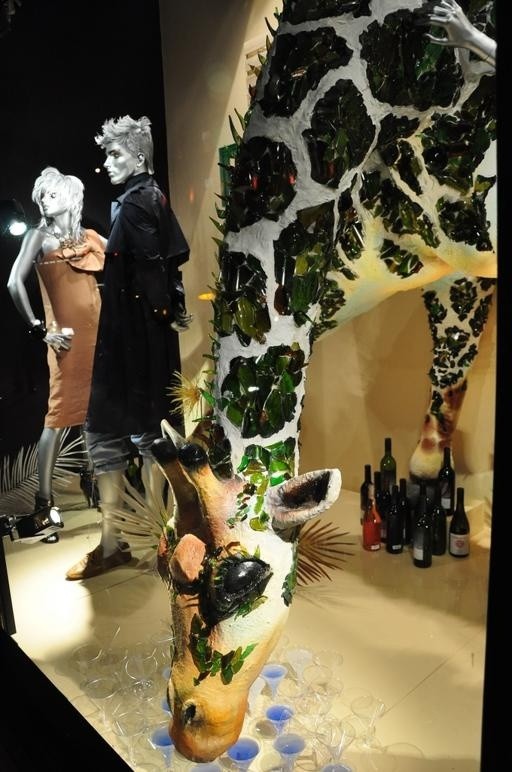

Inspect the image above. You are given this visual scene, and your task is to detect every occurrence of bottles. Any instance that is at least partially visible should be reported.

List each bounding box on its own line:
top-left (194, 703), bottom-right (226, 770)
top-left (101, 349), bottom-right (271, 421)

top-left (360, 438), bottom-right (473, 567)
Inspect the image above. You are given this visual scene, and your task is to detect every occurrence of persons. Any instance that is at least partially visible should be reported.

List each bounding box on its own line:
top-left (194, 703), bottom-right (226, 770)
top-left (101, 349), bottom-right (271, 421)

top-left (64, 115), bottom-right (189, 583)
top-left (5, 166), bottom-right (109, 542)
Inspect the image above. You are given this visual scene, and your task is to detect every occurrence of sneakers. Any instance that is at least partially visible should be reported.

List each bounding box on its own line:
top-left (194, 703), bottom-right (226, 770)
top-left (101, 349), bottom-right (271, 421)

top-left (66, 542), bottom-right (131, 579)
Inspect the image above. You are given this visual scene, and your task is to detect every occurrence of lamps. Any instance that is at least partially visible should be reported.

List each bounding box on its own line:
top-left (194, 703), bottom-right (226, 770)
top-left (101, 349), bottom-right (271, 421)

top-left (2, 506), bottom-right (63, 545)
top-left (8, 213), bottom-right (27, 237)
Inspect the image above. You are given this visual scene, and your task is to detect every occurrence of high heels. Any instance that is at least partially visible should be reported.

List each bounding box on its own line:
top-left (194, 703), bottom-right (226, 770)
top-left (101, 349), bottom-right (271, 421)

top-left (34, 491), bottom-right (59, 544)
top-left (80, 472), bottom-right (100, 508)
